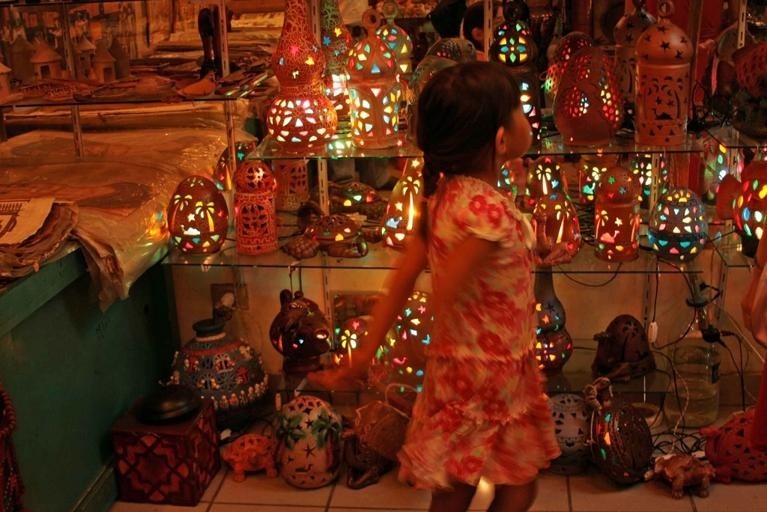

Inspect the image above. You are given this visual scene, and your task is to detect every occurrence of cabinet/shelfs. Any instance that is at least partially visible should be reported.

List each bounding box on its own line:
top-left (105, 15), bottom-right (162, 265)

top-left (1, 0), bottom-right (276, 170)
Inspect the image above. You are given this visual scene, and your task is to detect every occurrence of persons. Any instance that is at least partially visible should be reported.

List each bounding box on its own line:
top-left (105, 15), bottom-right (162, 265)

top-left (465, 0), bottom-right (505, 51)
top-left (308, 60), bottom-right (563, 512)
top-left (431, 0), bottom-right (468, 38)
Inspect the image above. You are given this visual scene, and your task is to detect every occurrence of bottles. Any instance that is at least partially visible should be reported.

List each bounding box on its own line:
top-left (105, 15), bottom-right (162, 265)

top-left (664, 295), bottom-right (720, 429)
